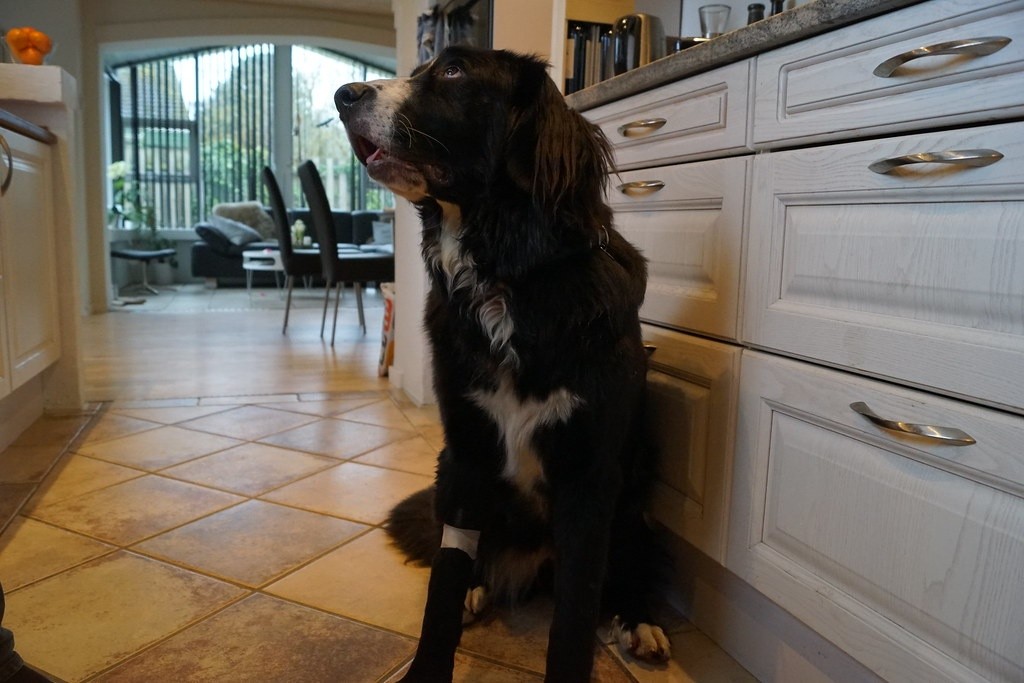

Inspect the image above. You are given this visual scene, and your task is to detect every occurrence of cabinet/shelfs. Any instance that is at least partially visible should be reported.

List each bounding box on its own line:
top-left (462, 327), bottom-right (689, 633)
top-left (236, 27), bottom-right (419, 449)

top-left (562, 0), bottom-right (1023, 683)
top-left (0, 108), bottom-right (61, 456)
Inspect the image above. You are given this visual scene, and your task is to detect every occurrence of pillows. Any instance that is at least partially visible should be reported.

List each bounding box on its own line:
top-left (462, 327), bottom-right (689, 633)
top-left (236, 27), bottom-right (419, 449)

top-left (194, 214), bottom-right (265, 256)
top-left (209, 201), bottom-right (276, 241)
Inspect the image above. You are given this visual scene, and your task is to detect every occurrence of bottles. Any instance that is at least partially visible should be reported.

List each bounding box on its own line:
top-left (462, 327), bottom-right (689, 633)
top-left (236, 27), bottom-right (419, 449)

top-left (770, 0), bottom-right (786, 19)
top-left (746, 4), bottom-right (764, 26)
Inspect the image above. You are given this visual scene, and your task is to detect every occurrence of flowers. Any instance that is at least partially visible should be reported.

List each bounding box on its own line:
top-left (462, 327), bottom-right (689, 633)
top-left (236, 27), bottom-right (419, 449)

top-left (107, 160), bottom-right (127, 209)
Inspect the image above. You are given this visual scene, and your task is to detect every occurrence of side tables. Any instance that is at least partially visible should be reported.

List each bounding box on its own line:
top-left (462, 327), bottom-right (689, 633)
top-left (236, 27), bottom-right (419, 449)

top-left (241, 247), bottom-right (286, 292)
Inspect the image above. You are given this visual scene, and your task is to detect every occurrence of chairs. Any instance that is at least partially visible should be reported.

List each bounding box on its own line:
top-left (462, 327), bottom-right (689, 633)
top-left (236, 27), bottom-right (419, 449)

top-left (298, 160), bottom-right (394, 347)
top-left (262, 166), bottom-right (367, 336)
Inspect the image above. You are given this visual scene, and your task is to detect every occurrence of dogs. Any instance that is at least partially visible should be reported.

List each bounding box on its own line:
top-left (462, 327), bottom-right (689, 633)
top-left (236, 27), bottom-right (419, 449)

top-left (333, 44), bottom-right (673, 683)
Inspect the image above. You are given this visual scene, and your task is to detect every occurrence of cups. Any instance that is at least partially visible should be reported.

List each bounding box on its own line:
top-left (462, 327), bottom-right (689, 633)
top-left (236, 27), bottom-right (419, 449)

top-left (698, 4), bottom-right (731, 38)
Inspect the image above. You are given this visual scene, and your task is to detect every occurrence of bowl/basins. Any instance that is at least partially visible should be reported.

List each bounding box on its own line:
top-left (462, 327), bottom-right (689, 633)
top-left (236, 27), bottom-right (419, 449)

top-left (1, 35), bottom-right (62, 65)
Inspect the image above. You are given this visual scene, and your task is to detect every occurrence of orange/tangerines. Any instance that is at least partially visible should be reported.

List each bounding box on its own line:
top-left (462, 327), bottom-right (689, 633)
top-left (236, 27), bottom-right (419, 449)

top-left (6, 27), bottom-right (54, 66)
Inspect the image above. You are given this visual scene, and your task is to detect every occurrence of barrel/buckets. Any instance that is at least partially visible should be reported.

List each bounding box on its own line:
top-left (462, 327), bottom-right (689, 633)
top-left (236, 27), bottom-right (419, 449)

top-left (611, 13), bottom-right (668, 77)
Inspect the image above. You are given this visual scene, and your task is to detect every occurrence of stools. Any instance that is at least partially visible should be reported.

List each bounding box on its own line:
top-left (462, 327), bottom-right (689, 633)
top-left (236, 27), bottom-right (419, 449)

top-left (111, 247), bottom-right (177, 296)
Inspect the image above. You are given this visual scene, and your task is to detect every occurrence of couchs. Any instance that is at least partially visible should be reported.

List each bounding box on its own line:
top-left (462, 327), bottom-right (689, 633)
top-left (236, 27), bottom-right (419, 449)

top-left (189, 204), bottom-right (395, 287)
